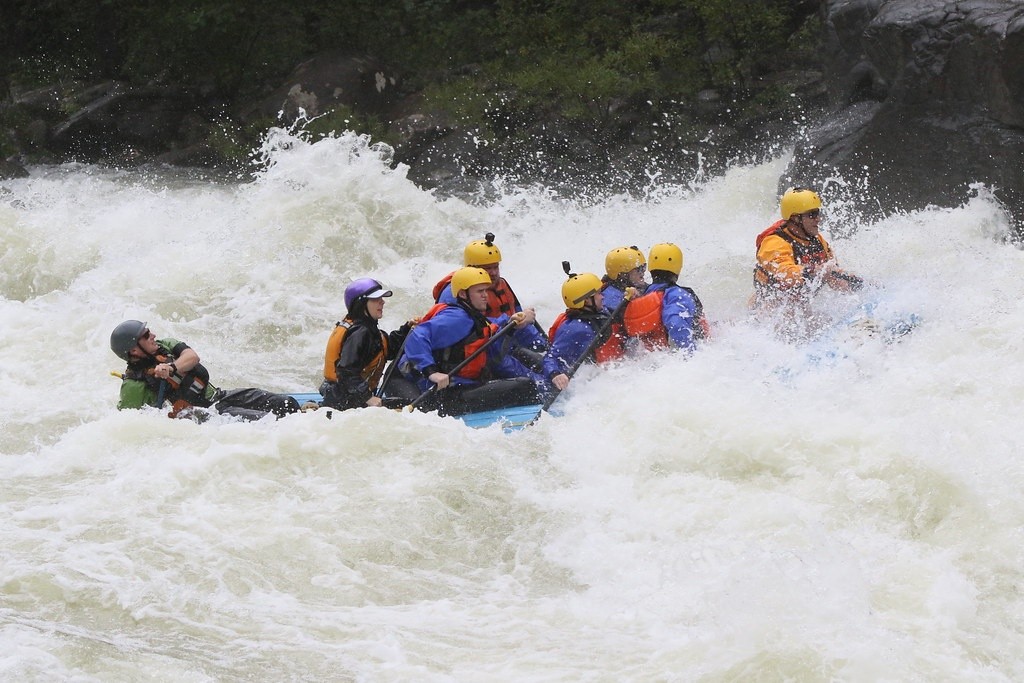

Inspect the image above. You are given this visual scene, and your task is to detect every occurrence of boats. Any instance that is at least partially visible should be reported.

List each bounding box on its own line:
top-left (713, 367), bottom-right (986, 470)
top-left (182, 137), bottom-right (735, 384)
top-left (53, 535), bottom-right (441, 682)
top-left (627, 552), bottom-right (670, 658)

top-left (753, 303), bottom-right (920, 378)
top-left (134, 391), bottom-right (562, 436)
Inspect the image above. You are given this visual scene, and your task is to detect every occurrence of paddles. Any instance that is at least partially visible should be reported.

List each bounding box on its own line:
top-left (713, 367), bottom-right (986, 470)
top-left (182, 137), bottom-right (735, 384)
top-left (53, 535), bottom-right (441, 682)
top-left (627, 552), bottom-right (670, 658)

top-left (528, 285), bottom-right (638, 427)
top-left (394, 306), bottom-right (527, 413)
top-left (145, 368), bottom-right (169, 410)
top-left (374, 314), bottom-right (422, 399)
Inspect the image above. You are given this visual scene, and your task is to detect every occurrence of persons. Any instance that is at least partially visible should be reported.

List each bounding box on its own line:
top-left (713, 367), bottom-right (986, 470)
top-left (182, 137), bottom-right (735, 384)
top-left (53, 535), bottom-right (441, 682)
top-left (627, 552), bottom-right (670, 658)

top-left (624, 242), bottom-right (704, 357)
top-left (433, 234), bottom-right (548, 370)
top-left (748, 187), bottom-right (861, 306)
top-left (398, 266), bottom-right (544, 391)
top-left (318, 277), bottom-right (422, 412)
top-left (539, 272), bottom-right (624, 398)
top-left (600, 247), bottom-right (649, 317)
top-left (111, 320), bottom-right (301, 422)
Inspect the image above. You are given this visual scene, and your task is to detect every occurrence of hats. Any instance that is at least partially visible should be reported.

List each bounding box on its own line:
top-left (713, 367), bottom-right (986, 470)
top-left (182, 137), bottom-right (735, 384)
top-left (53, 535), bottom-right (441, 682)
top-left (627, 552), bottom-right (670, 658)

top-left (365, 288), bottom-right (393, 298)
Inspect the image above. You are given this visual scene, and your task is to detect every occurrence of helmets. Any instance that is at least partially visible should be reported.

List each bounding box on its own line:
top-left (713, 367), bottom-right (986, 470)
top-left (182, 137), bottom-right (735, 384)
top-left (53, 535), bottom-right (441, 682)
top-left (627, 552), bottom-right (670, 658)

top-left (110, 320), bottom-right (147, 362)
top-left (344, 278), bottom-right (382, 313)
top-left (648, 242), bottom-right (683, 275)
top-left (781, 187), bottom-right (822, 221)
top-left (451, 266), bottom-right (492, 298)
top-left (561, 261), bottom-right (604, 309)
top-left (605, 246), bottom-right (646, 280)
top-left (464, 232), bottom-right (502, 267)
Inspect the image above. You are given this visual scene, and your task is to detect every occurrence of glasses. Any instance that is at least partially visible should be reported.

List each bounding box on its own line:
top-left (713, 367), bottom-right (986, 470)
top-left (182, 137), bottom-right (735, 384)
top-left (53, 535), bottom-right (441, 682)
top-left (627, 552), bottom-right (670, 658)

top-left (802, 209), bottom-right (820, 219)
top-left (137, 328), bottom-right (150, 340)
top-left (633, 263), bottom-right (646, 272)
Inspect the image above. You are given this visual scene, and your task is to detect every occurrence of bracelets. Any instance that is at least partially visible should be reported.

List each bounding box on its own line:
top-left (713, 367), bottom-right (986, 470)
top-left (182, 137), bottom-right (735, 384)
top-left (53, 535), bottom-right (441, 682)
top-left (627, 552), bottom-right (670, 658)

top-left (167, 362), bottom-right (177, 377)
